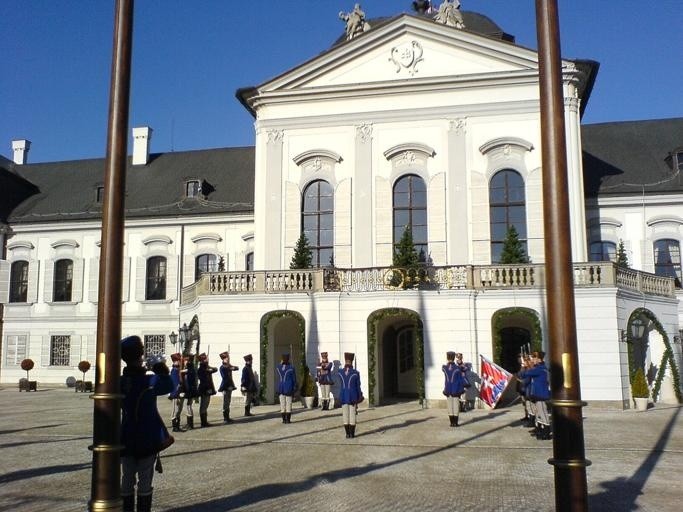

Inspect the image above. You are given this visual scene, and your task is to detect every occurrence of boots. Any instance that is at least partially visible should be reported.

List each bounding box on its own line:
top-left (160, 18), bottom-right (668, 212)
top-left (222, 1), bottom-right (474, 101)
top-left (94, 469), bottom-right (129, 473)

top-left (322, 399), bottom-right (330, 410)
top-left (120, 491), bottom-right (136, 512)
top-left (344, 424), bottom-right (357, 438)
top-left (245, 404), bottom-right (255, 416)
top-left (137, 488), bottom-right (153, 512)
top-left (281, 413), bottom-right (292, 424)
top-left (172, 416), bottom-right (187, 432)
top-left (449, 416), bottom-right (461, 427)
top-left (200, 412), bottom-right (213, 428)
top-left (182, 415), bottom-right (194, 431)
top-left (223, 412), bottom-right (233, 425)
top-left (458, 401), bottom-right (466, 412)
top-left (521, 413), bottom-right (552, 440)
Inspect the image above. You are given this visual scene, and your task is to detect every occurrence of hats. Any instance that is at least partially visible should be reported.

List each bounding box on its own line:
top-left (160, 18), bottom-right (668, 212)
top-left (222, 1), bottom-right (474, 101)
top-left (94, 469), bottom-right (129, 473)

top-left (455, 353), bottom-right (462, 356)
top-left (119, 336), bottom-right (144, 357)
top-left (321, 352), bottom-right (327, 356)
top-left (447, 352), bottom-right (455, 355)
top-left (220, 352), bottom-right (228, 358)
top-left (345, 352), bottom-right (354, 360)
top-left (244, 355), bottom-right (252, 360)
top-left (517, 352), bottom-right (545, 358)
top-left (283, 352), bottom-right (288, 355)
top-left (171, 353), bottom-right (206, 360)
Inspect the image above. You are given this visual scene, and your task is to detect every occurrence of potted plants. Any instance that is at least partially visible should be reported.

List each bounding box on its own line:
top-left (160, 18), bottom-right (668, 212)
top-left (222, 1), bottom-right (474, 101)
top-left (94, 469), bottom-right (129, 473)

top-left (302, 382), bottom-right (317, 410)
top-left (630, 366), bottom-right (652, 412)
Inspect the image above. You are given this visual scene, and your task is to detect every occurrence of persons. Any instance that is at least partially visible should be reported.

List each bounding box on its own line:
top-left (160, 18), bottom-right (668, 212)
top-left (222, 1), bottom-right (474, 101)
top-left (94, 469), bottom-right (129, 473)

top-left (441, 350), bottom-right (465, 428)
top-left (217, 351), bottom-right (239, 424)
top-left (239, 354), bottom-right (258, 419)
top-left (166, 351), bottom-right (186, 432)
top-left (179, 353), bottom-right (198, 430)
top-left (314, 350), bottom-right (334, 412)
top-left (274, 350), bottom-right (299, 424)
top-left (196, 352), bottom-right (217, 428)
top-left (116, 334), bottom-right (175, 512)
top-left (454, 353), bottom-right (471, 414)
top-left (338, 352), bottom-right (364, 439)
top-left (515, 343), bottom-right (552, 441)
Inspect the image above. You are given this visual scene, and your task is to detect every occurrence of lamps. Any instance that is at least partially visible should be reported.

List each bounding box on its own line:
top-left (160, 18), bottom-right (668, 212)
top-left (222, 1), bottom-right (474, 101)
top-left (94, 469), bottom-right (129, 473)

top-left (177, 323), bottom-right (199, 344)
top-left (169, 331), bottom-right (178, 346)
top-left (619, 315), bottom-right (646, 343)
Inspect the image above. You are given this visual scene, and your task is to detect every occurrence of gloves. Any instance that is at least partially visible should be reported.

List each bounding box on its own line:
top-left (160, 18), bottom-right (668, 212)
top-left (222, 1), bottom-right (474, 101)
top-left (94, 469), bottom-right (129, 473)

top-left (148, 352), bottom-right (167, 366)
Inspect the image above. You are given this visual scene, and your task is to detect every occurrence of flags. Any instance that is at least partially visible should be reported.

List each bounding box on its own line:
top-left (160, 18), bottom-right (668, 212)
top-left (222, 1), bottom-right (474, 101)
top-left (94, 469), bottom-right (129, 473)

top-left (476, 356), bottom-right (513, 410)
